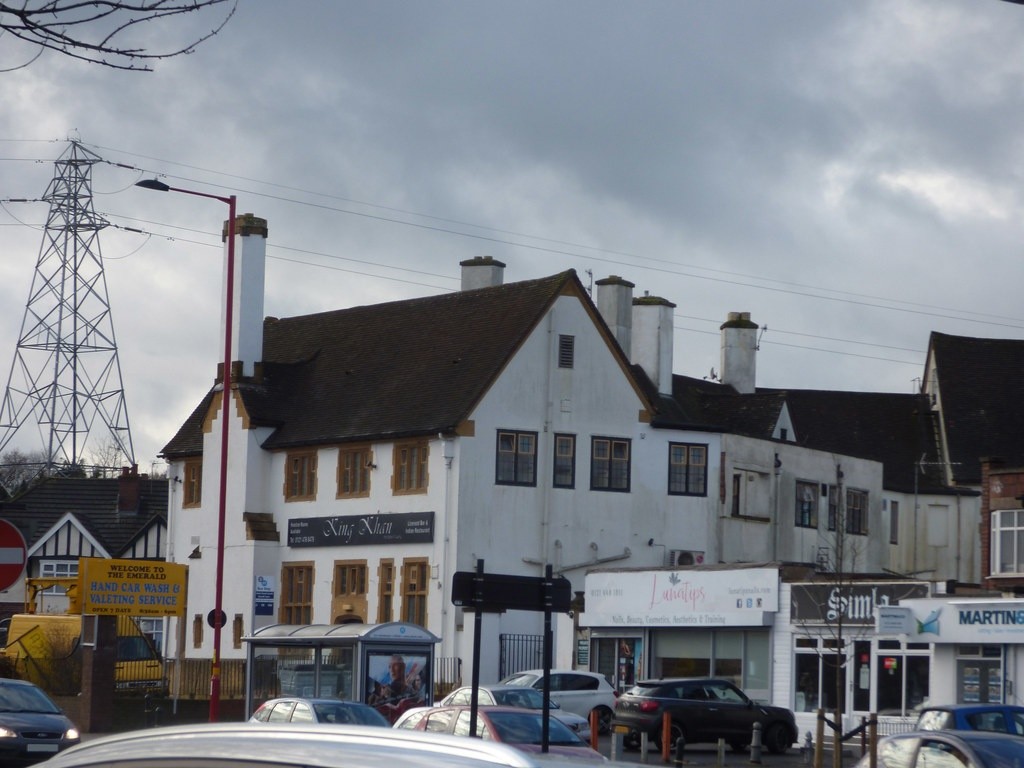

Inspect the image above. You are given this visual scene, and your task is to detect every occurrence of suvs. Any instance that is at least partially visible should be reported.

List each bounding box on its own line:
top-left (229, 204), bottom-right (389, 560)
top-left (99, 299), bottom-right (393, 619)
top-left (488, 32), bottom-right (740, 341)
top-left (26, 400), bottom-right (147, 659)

top-left (612, 676), bottom-right (799, 756)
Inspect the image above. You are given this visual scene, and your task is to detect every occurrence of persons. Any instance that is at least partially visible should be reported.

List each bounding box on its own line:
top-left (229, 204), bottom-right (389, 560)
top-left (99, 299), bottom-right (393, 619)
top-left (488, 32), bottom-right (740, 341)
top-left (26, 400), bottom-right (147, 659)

top-left (619, 639), bottom-right (630, 657)
top-left (368, 655), bottom-right (424, 704)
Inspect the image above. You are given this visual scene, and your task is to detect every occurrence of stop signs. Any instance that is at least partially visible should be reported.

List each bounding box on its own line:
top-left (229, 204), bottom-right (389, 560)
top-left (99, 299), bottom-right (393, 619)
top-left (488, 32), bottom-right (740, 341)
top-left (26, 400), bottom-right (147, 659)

top-left (0, 518), bottom-right (28, 591)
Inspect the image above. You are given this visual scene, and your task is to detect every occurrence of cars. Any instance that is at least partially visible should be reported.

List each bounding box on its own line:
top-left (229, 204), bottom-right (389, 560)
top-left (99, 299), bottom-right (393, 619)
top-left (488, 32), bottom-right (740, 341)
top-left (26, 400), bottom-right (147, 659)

top-left (392, 706), bottom-right (431, 729)
top-left (914, 702), bottom-right (1024, 754)
top-left (24, 719), bottom-right (668, 767)
top-left (246, 697), bottom-right (393, 729)
top-left (852, 731), bottom-right (1024, 768)
top-left (0, 677), bottom-right (81, 768)
top-left (432, 684), bottom-right (593, 745)
top-left (417, 705), bottom-right (608, 761)
top-left (499, 668), bottom-right (621, 737)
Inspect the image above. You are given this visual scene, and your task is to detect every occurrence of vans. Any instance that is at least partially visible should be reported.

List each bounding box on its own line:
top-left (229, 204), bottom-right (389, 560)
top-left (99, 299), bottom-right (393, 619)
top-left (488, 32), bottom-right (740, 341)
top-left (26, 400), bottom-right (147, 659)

top-left (5, 576), bottom-right (170, 728)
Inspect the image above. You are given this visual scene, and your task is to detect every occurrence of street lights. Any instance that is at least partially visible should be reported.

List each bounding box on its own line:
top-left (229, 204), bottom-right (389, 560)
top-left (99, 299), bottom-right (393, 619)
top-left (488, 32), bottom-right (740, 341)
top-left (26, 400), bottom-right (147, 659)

top-left (133, 176), bottom-right (237, 723)
